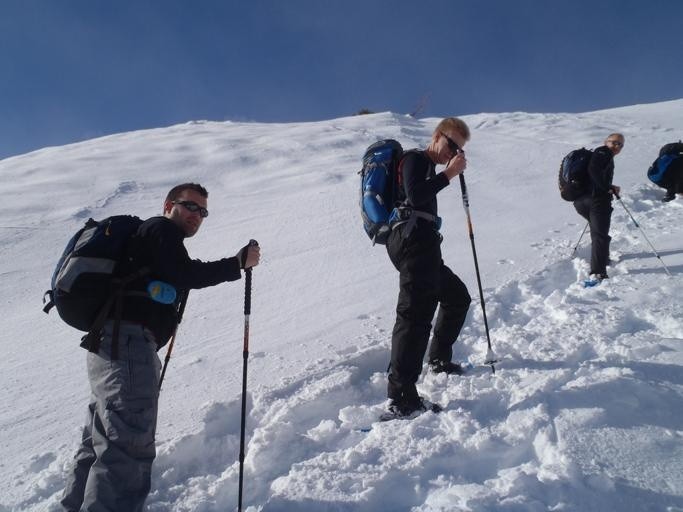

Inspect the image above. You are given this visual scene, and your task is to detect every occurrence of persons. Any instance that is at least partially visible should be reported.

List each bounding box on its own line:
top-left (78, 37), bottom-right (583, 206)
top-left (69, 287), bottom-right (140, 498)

top-left (574, 133), bottom-right (625, 281)
top-left (59, 183), bottom-right (261, 512)
top-left (660, 140), bottom-right (683, 201)
top-left (385, 118), bottom-right (471, 415)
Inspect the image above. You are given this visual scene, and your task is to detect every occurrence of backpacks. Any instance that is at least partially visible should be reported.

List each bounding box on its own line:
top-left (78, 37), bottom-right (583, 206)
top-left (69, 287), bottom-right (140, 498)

top-left (559, 149), bottom-right (595, 201)
top-left (51, 215), bottom-right (177, 331)
top-left (647, 151), bottom-right (683, 187)
top-left (361, 139), bottom-right (430, 244)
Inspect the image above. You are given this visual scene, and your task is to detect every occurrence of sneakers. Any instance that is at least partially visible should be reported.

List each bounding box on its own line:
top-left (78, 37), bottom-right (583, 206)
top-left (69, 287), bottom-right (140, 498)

top-left (590, 274), bottom-right (608, 281)
top-left (429, 358), bottom-right (471, 375)
top-left (383, 397), bottom-right (441, 420)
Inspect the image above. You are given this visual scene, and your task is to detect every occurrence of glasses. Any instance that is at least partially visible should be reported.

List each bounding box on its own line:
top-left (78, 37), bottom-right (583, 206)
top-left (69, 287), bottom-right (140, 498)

top-left (172, 200), bottom-right (208, 217)
top-left (440, 133), bottom-right (464, 155)
top-left (608, 140), bottom-right (623, 148)
